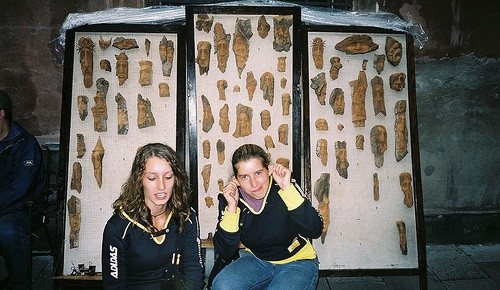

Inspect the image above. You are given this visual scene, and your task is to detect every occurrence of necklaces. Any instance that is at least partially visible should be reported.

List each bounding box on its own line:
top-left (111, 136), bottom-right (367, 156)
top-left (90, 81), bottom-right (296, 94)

top-left (152, 209), bottom-right (167, 217)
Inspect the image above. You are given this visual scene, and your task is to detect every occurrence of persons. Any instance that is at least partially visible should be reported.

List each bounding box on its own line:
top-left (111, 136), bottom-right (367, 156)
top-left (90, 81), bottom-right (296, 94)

top-left (0, 90), bottom-right (47, 290)
top-left (102, 142), bottom-right (207, 290)
top-left (210, 144), bottom-right (323, 290)
